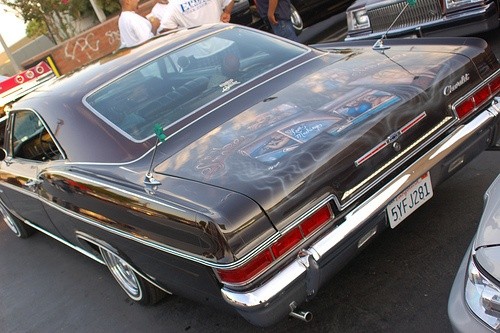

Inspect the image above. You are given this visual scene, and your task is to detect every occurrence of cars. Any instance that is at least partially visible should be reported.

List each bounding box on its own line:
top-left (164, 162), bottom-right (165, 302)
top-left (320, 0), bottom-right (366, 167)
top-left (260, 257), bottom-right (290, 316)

top-left (221, 0), bottom-right (500, 46)
top-left (0, 22), bottom-right (500, 325)
top-left (448, 173), bottom-right (500, 333)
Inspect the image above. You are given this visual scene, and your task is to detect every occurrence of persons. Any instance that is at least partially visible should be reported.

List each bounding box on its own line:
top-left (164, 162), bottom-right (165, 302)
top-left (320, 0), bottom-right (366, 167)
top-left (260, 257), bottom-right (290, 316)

top-left (118, 71), bottom-right (181, 106)
top-left (117, 0), bottom-right (299, 49)
